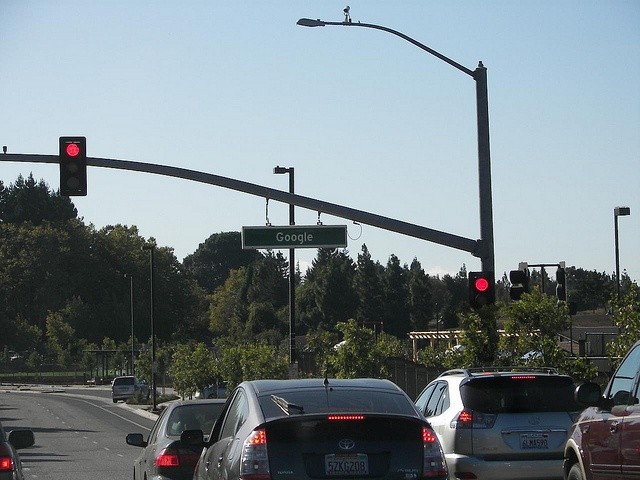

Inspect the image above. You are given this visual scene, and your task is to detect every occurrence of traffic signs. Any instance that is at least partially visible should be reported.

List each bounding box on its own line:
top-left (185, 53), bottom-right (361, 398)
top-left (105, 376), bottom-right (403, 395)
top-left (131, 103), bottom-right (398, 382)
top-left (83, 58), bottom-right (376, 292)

top-left (242, 225), bottom-right (347, 249)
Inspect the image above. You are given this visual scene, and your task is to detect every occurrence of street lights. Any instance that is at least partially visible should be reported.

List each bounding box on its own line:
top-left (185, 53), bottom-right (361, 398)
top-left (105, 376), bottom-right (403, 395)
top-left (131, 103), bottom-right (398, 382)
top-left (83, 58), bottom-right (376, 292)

top-left (296, 18), bottom-right (495, 316)
top-left (614, 208), bottom-right (630, 300)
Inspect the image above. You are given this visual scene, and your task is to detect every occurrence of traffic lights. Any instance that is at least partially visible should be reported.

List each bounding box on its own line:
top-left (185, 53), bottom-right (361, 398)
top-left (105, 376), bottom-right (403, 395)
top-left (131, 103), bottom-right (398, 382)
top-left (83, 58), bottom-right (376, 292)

top-left (510, 270), bottom-right (526, 300)
top-left (556, 268), bottom-right (566, 301)
top-left (469, 272), bottom-right (492, 309)
top-left (59, 136), bottom-right (87, 196)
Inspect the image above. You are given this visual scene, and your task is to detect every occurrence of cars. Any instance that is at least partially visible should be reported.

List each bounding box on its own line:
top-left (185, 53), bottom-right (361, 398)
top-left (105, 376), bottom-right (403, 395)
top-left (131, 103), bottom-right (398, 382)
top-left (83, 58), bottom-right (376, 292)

top-left (194, 381), bottom-right (228, 398)
top-left (0, 422), bottom-right (35, 480)
top-left (522, 347), bottom-right (578, 362)
top-left (562, 339), bottom-right (639, 480)
top-left (125, 399), bottom-right (228, 480)
top-left (180, 379), bottom-right (450, 480)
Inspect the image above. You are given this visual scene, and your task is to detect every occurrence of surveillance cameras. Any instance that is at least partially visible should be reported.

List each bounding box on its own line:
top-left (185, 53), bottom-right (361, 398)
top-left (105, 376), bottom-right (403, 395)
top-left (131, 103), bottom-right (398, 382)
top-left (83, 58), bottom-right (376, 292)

top-left (343, 5), bottom-right (350, 20)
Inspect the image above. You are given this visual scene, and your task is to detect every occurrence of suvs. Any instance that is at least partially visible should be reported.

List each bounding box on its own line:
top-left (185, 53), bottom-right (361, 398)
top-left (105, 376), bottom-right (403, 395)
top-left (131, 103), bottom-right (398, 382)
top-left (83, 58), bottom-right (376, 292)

top-left (415, 368), bottom-right (590, 480)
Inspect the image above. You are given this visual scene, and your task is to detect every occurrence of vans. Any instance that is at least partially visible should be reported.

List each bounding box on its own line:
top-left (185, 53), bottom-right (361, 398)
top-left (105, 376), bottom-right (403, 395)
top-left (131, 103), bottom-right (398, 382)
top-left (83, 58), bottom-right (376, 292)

top-left (112, 376), bottom-right (150, 403)
